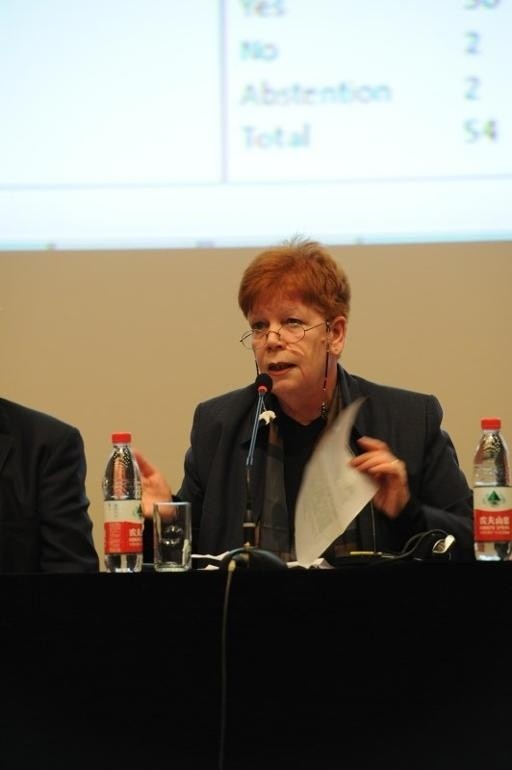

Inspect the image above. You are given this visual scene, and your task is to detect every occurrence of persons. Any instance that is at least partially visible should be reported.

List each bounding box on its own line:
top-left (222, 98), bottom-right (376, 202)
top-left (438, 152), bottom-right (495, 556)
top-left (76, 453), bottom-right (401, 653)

top-left (0, 398), bottom-right (101, 580)
top-left (108, 235), bottom-right (475, 562)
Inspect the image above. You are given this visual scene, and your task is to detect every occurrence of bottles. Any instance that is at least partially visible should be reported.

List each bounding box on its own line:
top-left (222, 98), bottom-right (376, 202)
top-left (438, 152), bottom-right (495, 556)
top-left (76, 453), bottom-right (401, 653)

top-left (101, 430), bottom-right (145, 577)
top-left (471, 414), bottom-right (512, 563)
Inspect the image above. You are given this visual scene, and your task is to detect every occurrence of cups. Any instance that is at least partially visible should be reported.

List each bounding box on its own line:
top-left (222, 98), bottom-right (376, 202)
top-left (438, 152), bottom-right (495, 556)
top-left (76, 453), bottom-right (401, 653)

top-left (152, 501), bottom-right (194, 571)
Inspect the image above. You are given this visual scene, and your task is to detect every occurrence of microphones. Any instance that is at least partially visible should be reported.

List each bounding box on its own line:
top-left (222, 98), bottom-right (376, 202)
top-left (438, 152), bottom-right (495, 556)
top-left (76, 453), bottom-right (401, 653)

top-left (220, 372), bottom-right (289, 571)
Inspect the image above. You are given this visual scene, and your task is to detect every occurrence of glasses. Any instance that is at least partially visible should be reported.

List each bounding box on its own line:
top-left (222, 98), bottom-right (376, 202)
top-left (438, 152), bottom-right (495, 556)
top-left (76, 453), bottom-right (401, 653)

top-left (240, 321), bottom-right (327, 349)
